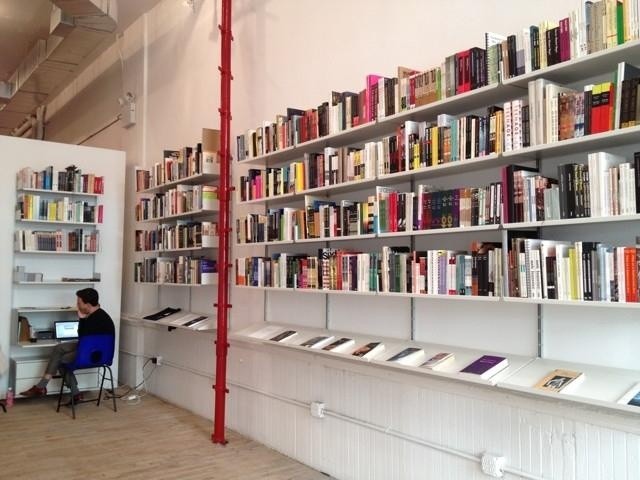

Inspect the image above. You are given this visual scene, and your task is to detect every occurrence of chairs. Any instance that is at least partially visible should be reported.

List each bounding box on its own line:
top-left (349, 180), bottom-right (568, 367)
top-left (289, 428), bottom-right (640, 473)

top-left (56, 334), bottom-right (118, 418)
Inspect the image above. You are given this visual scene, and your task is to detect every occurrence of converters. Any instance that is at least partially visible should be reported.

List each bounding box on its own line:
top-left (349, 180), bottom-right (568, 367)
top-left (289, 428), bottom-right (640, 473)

top-left (128, 395), bottom-right (137, 400)
top-left (152, 357), bottom-right (157, 364)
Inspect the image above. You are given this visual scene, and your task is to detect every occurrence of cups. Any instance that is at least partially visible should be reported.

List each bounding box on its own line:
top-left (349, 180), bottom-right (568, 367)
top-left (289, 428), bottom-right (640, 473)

top-left (29, 327), bottom-right (39, 343)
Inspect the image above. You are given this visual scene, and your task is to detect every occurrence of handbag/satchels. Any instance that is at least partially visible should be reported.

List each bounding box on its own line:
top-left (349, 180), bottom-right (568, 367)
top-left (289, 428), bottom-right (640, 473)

top-left (18, 316), bottom-right (30, 341)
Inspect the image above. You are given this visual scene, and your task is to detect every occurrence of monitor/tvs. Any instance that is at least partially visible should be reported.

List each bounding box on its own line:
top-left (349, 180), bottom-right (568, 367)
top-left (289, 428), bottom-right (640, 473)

top-left (54, 321), bottom-right (80, 341)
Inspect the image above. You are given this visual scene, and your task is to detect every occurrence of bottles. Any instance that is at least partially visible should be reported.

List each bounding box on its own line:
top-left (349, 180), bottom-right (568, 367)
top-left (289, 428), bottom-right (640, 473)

top-left (6, 387), bottom-right (14, 407)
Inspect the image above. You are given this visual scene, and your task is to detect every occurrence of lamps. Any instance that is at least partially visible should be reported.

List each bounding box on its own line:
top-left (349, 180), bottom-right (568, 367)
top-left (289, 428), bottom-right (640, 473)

top-left (117, 91), bottom-right (136, 129)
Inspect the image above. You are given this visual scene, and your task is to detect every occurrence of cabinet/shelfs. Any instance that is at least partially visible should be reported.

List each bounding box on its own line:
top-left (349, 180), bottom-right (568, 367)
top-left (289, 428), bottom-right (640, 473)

top-left (9, 179), bottom-right (102, 398)
top-left (134, 37), bottom-right (639, 412)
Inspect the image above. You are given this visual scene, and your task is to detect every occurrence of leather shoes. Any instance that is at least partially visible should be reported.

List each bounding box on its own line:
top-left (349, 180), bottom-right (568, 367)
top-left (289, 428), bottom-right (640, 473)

top-left (71, 393), bottom-right (83, 402)
top-left (20, 385), bottom-right (47, 398)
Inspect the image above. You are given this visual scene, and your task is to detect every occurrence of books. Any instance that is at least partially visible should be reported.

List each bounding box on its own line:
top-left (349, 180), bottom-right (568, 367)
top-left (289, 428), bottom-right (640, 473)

top-left (460, 356), bottom-right (509, 382)
top-left (142, 307), bottom-right (209, 327)
top-left (617, 384), bottom-right (639, 406)
top-left (14, 165), bottom-right (104, 252)
top-left (299, 336), bottom-right (333, 348)
top-left (420, 352), bottom-right (454, 372)
top-left (385, 348), bottom-right (424, 364)
top-left (323, 338), bottom-right (354, 351)
top-left (270, 330), bottom-right (296, 342)
top-left (230, 1), bottom-right (639, 304)
top-left (535, 368), bottom-right (583, 392)
top-left (352, 342), bottom-right (384, 359)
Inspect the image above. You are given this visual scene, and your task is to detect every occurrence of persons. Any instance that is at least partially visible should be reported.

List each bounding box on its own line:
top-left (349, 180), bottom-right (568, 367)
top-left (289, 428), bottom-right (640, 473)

top-left (18, 288), bottom-right (115, 408)
top-left (134, 127), bottom-right (221, 284)
